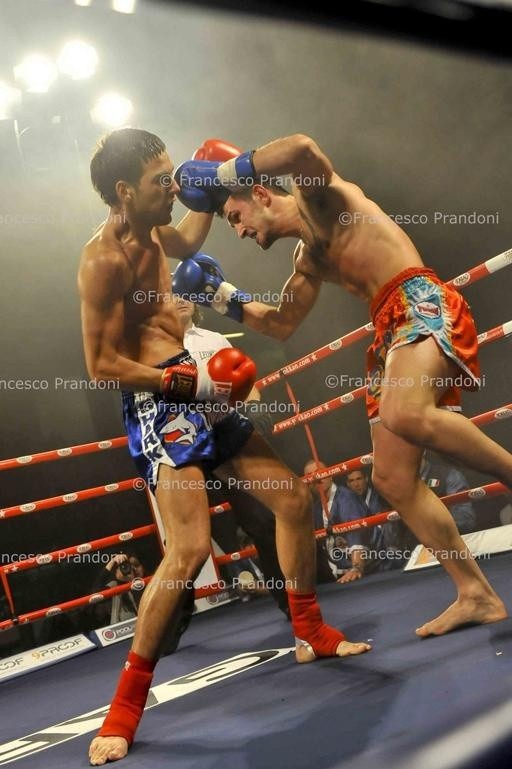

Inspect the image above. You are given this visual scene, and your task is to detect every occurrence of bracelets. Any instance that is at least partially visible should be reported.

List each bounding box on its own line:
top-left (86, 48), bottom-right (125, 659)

top-left (111, 557), bottom-right (116, 566)
top-left (350, 564), bottom-right (365, 574)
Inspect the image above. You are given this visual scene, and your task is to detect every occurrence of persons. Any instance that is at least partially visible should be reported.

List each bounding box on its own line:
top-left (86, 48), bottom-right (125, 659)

top-left (345, 462), bottom-right (402, 566)
top-left (166, 128), bottom-right (512, 642)
top-left (391, 440), bottom-right (478, 566)
top-left (212, 518), bottom-right (270, 599)
top-left (300, 456), bottom-right (391, 585)
top-left (84, 546), bottom-right (154, 621)
top-left (74, 127), bottom-right (374, 768)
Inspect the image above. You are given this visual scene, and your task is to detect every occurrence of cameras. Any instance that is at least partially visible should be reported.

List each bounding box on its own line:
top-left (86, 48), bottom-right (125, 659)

top-left (119, 559), bottom-right (131, 577)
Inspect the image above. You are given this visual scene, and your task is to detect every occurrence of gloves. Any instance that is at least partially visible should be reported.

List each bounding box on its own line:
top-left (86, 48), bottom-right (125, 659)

top-left (172, 149), bottom-right (260, 214)
top-left (190, 139), bottom-right (245, 163)
top-left (169, 251), bottom-right (256, 324)
top-left (160, 346), bottom-right (259, 408)
